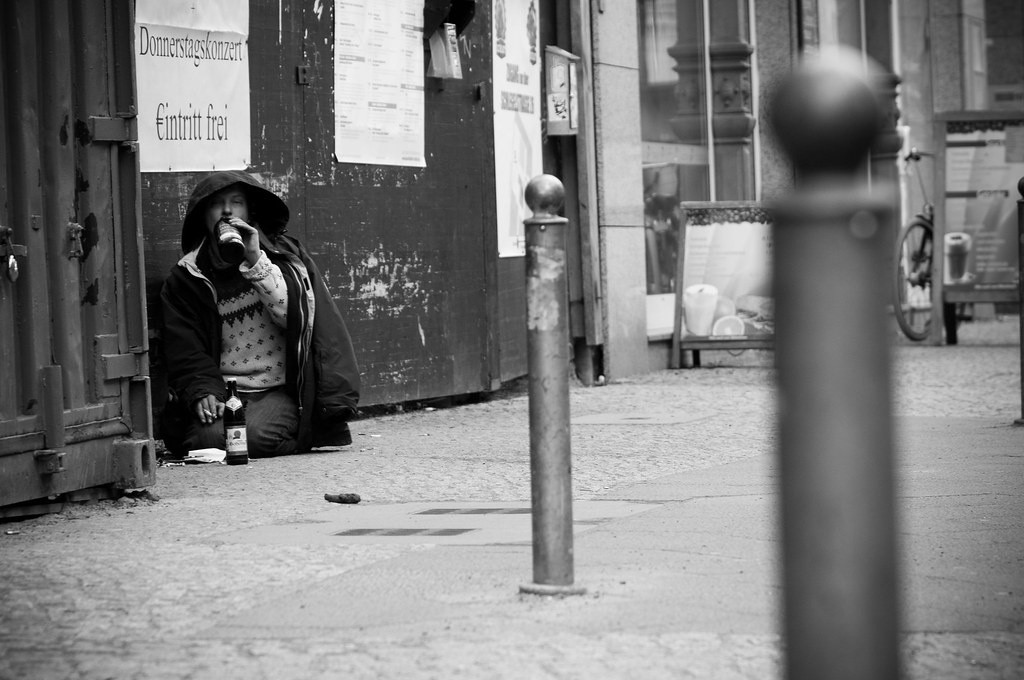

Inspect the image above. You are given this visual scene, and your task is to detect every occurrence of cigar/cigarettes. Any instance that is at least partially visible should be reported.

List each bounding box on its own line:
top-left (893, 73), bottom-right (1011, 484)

top-left (203, 409), bottom-right (217, 418)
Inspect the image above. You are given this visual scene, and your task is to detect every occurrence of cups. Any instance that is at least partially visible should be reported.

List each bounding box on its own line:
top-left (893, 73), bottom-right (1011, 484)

top-left (944, 232), bottom-right (972, 279)
top-left (684, 284), bottom-right (718, 337)
top-left (712, 315), bottom-right (745, 336)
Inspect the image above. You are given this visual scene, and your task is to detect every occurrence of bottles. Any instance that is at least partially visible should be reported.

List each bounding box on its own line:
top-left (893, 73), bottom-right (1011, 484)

top-left (213, 217), bottom-right (248, 263)
top-left (223, 378), bottom-right (250, 466)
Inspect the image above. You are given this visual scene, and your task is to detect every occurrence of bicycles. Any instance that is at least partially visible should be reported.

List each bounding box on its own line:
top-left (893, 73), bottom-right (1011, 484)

top-left (895, 149), bottom-right (966, 341)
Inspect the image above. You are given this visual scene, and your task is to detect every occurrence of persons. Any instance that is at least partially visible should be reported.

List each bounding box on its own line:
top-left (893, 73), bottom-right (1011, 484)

top-left (153, 171), bottom-right (361, 457)
